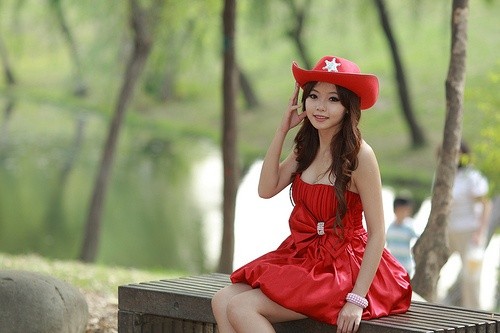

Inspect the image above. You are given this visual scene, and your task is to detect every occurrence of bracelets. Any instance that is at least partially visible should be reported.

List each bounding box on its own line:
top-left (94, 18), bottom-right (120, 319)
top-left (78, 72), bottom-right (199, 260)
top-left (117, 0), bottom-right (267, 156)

top-left (346, 293), bottom-right (369, 308)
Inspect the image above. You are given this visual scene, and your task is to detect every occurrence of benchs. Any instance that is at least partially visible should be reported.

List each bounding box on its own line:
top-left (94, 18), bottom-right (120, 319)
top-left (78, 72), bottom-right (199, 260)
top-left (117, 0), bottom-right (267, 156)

top-left (118, 273), bottom-right (500, 333)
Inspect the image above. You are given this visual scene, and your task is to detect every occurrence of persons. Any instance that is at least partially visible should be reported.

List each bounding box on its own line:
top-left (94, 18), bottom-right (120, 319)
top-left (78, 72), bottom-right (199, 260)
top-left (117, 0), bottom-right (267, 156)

top-left (386, 191), bottom-right (419, 275)
top-left (211, 55), bottom-right (413, 333)
top-left (431, 139), bottom-right (492, 311)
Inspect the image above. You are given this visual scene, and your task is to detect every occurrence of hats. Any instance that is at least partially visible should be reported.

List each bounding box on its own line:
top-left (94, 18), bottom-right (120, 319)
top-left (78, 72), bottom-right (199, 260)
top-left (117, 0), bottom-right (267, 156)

top-left (292, 56), bottom-right (379, 110)
